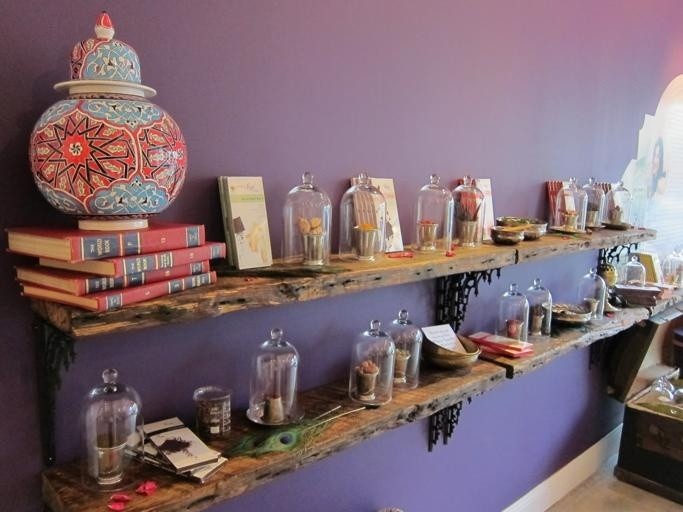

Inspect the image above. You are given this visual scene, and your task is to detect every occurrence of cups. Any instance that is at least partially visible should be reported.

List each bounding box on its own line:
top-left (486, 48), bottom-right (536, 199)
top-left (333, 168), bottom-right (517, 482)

top-left (457, 221), bottom-right (477, 248)
top-left (416, 223), bottom-right (439, 251)
top-left (392, 354), bottom-right (411, 384)
top-left (531, 314), bottom-right (544, 336)
top-left (354, 366), bottom-right (379, 401)
top-left (351, 227), bottom-right (377, 261)
top-left (263, 394), bottom-right (283, 424)
top-left (89, 431), bottom-right (128, 482)
top-left (191, 385), bottom-right (233, 439)
top-left (301, 234), bottom-right (324, 266)
top-left (504, 319), bottom-right (524, 341)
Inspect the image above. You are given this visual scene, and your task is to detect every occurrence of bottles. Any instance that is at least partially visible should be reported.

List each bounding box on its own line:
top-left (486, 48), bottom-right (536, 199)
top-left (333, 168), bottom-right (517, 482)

top-left (494, 282), bottom-right (529, 344)
top-left (525, 277), bottom-right (552, 340)
top-left (388, 308), bottom-right (423, 391)
top-left (336, 171), bottom-right (386, 265)
top-left (577, 246), bottom-right (682, 322)
top-left (553, 176), bottom-right (633, 233)
top-left (347, 319), bottom-right (392, 405)
top-left (80, 367), bottom-right (145, 492)
top-left (412, 173), bottom-right (453, 254)
top-left (279, 170), bottom-right (332, 268)
top-left (248, 325), bottom-right (299, 425)
top-left (451, 174), bottom-right (485, 250)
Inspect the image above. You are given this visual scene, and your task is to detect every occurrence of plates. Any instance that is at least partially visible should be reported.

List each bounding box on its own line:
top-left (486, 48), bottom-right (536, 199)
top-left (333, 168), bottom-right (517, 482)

top-left (540, 301), bottom-right (592, 328)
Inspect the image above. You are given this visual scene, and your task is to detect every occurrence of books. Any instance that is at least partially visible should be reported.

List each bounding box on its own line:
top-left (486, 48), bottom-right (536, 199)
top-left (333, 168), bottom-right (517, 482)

top-left (222, 175), bottom-right (273, 272)
top-left (216, 173), bottom-right (234, 267)
top-left (1, 224), bottom-right (208, 265)
top-left (12, 260), bottom-right (211, 298)
top-left (125, 432), bottom-right (222, 476)
top-left (628, 252), bottom-right (663, 284)
top-left (351, 176), bottom-right (404, 254)
top-left (136, 416), bottom-right (219, 473)
top-left (595, 182), bottom-right (621, 220)
top-left (545, 179), bottom-right (575, 225)
top-left (37, 240), bottom-right (225, 280)
top-left (457, 176), bottom-right (495, 241)
top-left (17, 268), bottom-right (218, 315)
top-left (122, 447), bottom-right (227, 485)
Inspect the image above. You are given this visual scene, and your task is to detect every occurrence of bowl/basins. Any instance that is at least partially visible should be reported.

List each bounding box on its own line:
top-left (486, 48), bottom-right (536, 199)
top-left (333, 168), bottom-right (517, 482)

top-left (422, 334), bottom-right (482, 369)
top-left (488, 225), bottom-right (524, 245)
top-left (495, 216), bottom-right (548, 240)
